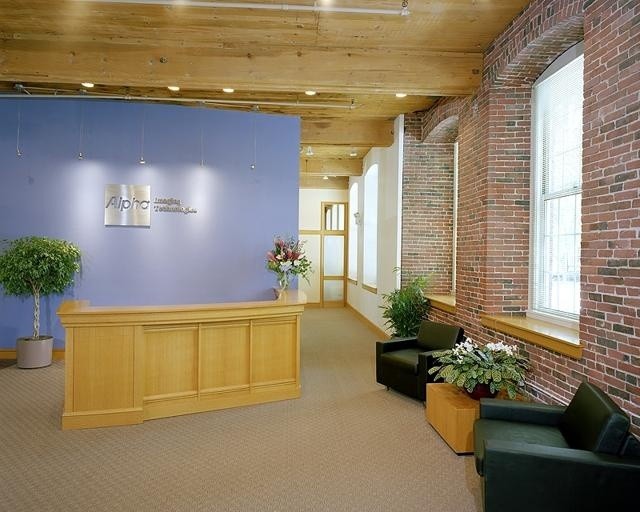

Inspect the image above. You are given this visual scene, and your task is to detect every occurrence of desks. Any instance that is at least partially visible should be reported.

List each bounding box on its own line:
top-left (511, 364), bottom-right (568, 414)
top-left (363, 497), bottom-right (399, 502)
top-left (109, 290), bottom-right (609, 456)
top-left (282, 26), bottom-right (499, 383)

top-left (423, 380), bottom-right (534, 456)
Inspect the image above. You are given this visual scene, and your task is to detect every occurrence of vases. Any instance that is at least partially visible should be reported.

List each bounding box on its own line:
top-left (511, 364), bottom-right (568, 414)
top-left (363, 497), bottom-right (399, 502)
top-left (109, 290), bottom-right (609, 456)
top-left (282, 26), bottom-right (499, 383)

top-left (277, 273), bottom-right (292, 290)
top-left (463, 377), bottom-right (501, 402)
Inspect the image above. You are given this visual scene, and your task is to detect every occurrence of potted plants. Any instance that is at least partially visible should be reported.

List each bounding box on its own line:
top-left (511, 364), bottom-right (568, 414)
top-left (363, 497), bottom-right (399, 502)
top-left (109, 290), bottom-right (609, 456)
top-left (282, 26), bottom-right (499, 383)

top-left (0, 237), bottom-right (81, 369)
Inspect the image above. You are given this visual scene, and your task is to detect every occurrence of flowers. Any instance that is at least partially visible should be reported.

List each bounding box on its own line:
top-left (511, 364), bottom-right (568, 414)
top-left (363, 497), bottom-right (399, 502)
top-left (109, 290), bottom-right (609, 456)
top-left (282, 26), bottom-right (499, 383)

top-left (426, 333), bottom-right (529, 402)
top-left (263, 236), bottom-right (313, 286)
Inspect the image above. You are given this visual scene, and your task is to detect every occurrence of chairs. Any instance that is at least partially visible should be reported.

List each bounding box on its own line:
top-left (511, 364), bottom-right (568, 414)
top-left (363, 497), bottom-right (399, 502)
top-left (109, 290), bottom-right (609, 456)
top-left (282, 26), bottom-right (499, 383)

top-left (471, 381), bottom-right (640, 512)
top-left (375, 319), bottom-right (467, 408)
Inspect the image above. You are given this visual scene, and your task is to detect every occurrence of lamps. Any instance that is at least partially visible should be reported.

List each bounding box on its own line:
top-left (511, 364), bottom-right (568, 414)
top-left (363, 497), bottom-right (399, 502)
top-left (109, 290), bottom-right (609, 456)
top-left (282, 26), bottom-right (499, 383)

top-left (13, 81), bottom-right (263, 172)
top-left (306, 145), bottom-right (314, 156)
